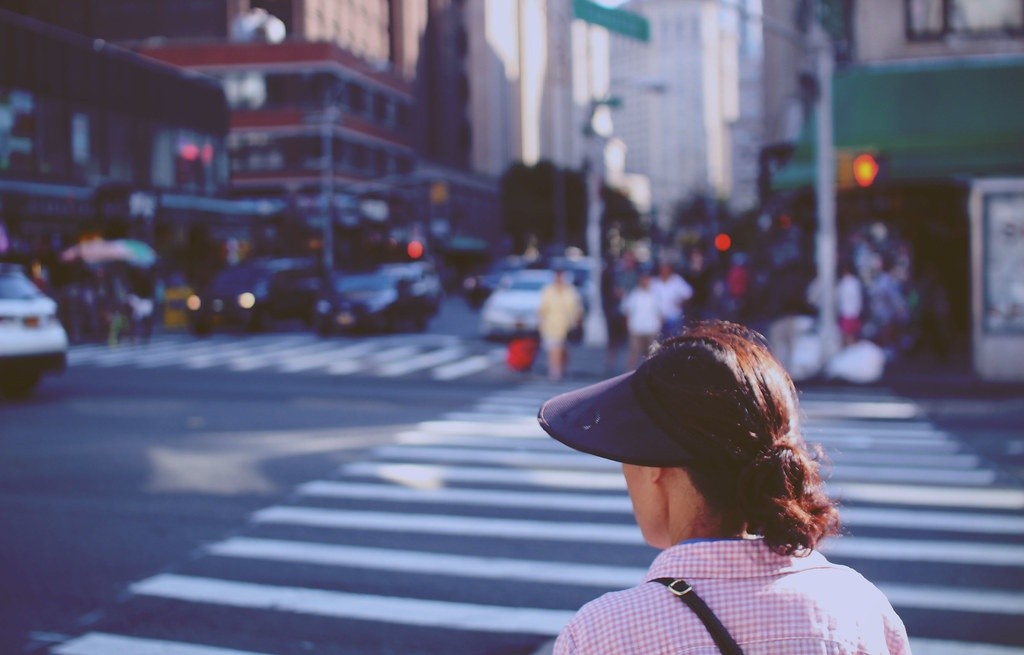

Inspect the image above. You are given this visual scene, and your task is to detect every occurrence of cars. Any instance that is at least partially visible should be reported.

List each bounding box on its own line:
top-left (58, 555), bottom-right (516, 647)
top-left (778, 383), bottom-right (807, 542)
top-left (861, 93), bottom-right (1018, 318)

top-left (0, 262), bottom-right (69, 402)
top-left (182, 242), bottom-right (600, 343)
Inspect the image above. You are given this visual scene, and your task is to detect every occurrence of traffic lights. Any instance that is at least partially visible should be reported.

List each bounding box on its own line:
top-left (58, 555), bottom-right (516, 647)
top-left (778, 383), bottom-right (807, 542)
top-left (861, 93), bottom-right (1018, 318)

top-left (836, 144), bottom-right (885, 195)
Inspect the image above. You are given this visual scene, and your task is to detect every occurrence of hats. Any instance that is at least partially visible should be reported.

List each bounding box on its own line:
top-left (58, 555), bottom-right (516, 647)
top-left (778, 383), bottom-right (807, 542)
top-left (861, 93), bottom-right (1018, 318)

top-left (538, 356), bottom-right (799, 468)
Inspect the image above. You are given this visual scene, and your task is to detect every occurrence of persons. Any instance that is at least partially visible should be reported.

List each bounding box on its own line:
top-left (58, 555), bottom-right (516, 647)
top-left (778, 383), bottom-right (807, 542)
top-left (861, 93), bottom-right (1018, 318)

top-left (54, 272), bottom-right (151, 346)
top-left (536, 220), bottom-right (917, 380)
top-left (537, 321), bottom-right (914, 655)
